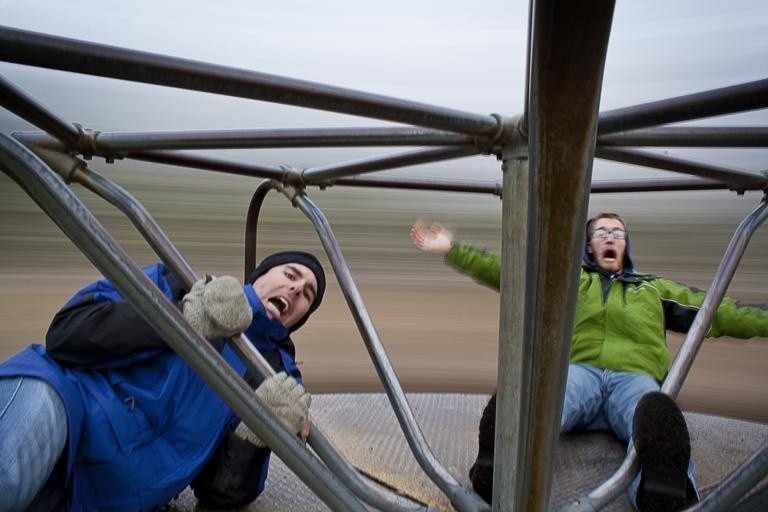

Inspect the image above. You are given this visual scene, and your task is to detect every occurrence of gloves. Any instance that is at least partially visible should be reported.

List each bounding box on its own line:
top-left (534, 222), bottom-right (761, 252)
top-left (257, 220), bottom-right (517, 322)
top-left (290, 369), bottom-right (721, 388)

top-left (182, 274), bottom-right (254, 340)
top-left (235, 371), bottom-right (312, 448)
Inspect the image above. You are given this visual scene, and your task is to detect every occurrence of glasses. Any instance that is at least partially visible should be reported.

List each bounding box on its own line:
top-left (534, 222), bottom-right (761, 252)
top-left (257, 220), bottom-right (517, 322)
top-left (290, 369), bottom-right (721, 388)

top-left (588, 228), bottom-right (628, 241)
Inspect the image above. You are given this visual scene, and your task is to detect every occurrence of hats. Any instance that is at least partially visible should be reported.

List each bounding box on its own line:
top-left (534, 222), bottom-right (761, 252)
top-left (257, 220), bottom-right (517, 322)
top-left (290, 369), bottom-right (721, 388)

top-left (248, 251), bottom-right (326, 334)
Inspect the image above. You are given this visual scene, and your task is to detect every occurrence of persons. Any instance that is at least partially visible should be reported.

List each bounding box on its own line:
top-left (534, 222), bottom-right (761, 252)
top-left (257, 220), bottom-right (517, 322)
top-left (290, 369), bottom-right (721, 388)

top-left (411, 212), bottom-right (768, 511)
top-left (0, 251), bottom-right (326, 512)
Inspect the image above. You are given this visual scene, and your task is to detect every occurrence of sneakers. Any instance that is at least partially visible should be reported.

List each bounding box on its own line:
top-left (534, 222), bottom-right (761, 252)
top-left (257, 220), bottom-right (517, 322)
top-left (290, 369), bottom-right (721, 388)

top-left (632, 391), bottom-right (692, 512)
top-left (469, 394), bottom-right (497, 504)
